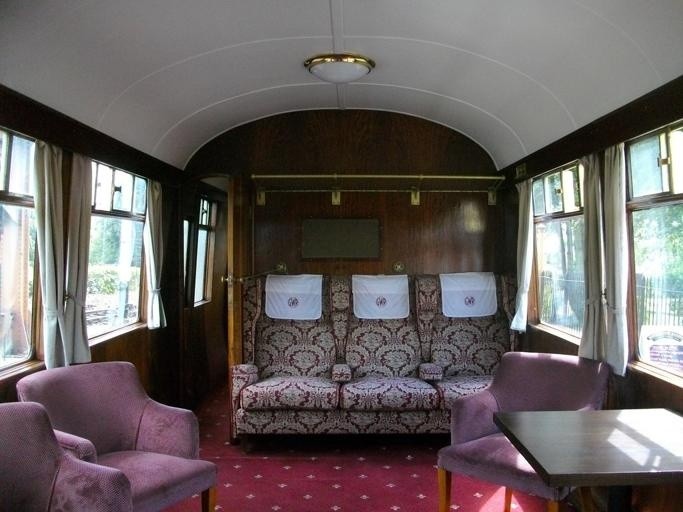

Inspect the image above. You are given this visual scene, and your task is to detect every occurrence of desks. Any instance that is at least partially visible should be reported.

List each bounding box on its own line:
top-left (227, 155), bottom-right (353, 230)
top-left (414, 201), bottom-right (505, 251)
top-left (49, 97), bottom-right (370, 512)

top-left (491, 407), bottom-right (682, 511)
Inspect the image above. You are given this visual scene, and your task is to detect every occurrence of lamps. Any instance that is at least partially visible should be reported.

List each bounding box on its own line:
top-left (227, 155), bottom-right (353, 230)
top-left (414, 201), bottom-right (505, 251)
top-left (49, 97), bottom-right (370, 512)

top-left (303, 50), bottom-right (377, 86)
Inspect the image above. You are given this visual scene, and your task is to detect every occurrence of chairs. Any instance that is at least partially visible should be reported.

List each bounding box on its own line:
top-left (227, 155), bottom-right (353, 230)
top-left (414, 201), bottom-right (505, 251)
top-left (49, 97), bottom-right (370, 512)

top-left (232, 274), bottom-right (342, 437)
top-left (414, 268), bottom-right (525, 436)
top-left (434, 351), bottom-right (612, 512)
top-left (343, 271), bottom-right (435, 437)
top-left (0, 360), bottom-right (216, 511)
